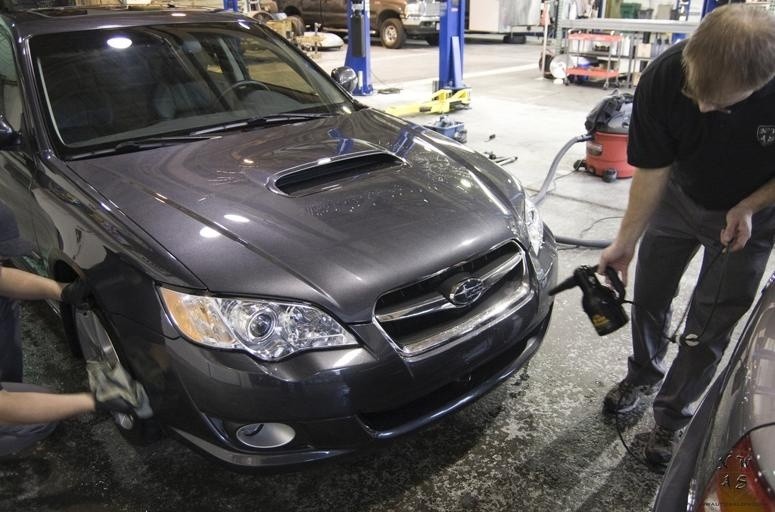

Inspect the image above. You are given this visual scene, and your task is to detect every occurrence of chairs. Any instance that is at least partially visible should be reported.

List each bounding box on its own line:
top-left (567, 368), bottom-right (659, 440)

top-left (149, 52), bottom-right (243, 124)
top-left (43, 59), bottom-right (116, 146)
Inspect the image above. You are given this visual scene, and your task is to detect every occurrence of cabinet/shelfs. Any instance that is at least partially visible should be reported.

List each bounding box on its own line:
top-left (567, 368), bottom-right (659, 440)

top-left (563, 29), bottom-right (624, 91)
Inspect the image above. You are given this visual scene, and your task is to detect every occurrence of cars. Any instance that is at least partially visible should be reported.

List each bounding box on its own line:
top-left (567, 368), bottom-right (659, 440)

top-left (0, 5), bottom-right (560, 479)
top-left (247, 0), bottom-right (447, 50)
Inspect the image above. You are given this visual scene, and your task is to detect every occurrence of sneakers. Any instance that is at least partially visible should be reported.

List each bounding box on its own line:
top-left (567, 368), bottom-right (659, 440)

top-left (642, 420), bottom-right (681, 466)
top-left (603, 374), bottom-right (664, 412)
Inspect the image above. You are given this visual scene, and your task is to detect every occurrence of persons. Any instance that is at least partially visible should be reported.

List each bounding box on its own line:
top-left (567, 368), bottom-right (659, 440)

top-left (0, 197), bottom-right (134, 458)
top-left (598, 2), bottom-right (775, 463)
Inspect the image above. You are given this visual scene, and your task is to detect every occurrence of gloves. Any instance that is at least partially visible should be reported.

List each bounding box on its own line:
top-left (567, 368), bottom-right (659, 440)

top-left (91, 387), bottom-right (137, 416)
top-left (58, 277), bottom-right (96, 311)
